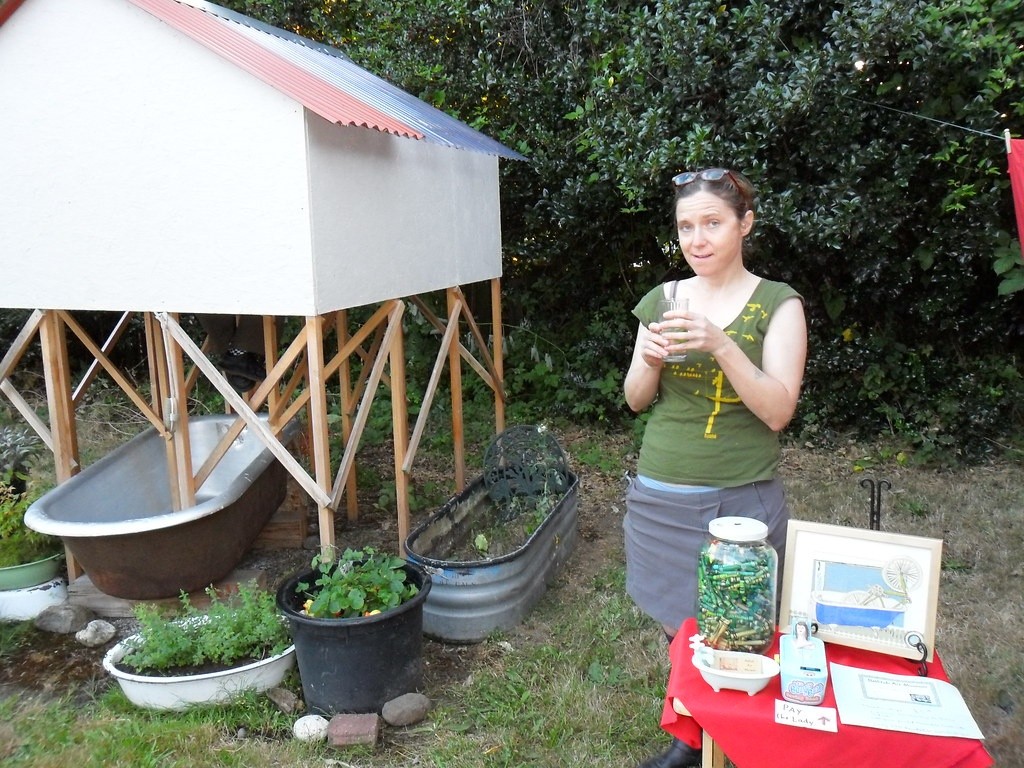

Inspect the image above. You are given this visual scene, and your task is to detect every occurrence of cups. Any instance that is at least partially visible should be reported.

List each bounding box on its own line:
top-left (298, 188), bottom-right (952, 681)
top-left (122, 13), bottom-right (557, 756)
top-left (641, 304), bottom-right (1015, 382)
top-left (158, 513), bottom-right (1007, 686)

top-left (658, 298), bottom-right (689, 363)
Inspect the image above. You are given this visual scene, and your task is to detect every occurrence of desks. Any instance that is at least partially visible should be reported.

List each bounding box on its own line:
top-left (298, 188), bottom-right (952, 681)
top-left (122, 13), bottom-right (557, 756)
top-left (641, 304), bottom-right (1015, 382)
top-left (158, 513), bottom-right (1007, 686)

top-left (659, 618), bottom-right (995, 768)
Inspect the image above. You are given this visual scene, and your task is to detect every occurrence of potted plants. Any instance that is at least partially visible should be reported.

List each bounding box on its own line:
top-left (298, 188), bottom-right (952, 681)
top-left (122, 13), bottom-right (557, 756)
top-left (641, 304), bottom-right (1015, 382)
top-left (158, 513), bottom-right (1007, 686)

top-left (402, 423), bottom-right (580, 645)
top-left (0, 426), bottom-right (46, 501)
top-left (276, 545), bottom-right (432, 718)
top-left (0, 464), bottom-right (66, 592)
top-left (103, 578), bottom-right (297, 715)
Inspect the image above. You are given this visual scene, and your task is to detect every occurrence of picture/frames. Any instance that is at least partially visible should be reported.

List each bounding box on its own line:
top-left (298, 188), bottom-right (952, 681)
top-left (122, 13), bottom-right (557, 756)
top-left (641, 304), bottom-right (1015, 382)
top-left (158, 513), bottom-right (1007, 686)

top-left (778, 518), bottom-right (944, 663)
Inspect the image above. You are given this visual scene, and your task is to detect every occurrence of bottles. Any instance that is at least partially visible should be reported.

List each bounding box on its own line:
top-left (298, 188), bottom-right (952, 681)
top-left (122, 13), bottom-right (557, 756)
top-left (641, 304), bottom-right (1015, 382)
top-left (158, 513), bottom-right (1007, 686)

top-left (696, 516), bottom-right (778, 655)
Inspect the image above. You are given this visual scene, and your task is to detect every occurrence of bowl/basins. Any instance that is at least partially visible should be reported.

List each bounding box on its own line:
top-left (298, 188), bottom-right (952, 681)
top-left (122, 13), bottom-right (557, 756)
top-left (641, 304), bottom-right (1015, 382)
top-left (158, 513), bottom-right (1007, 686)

top-left (692, 650), bottom-right (780, 697)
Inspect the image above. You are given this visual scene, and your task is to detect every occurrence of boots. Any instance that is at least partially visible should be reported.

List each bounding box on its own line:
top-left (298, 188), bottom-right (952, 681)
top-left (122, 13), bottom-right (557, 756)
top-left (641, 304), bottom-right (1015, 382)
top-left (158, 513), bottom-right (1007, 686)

top-left (635, 625), bottom-right (702, 768)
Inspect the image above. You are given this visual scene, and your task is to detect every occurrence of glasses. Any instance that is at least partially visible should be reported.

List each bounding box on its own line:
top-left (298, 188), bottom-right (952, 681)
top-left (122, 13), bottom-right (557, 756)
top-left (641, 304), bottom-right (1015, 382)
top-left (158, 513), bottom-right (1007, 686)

top-left (670, 168), bottom-right (743, 196)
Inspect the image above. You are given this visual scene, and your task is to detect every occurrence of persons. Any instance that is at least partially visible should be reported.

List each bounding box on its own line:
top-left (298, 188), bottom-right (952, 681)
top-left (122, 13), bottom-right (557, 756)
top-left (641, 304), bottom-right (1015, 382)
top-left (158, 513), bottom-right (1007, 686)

top-left (623, 168), bottom-right (807, 768)
top-left (195, 314), bottom-right (266, 393)
